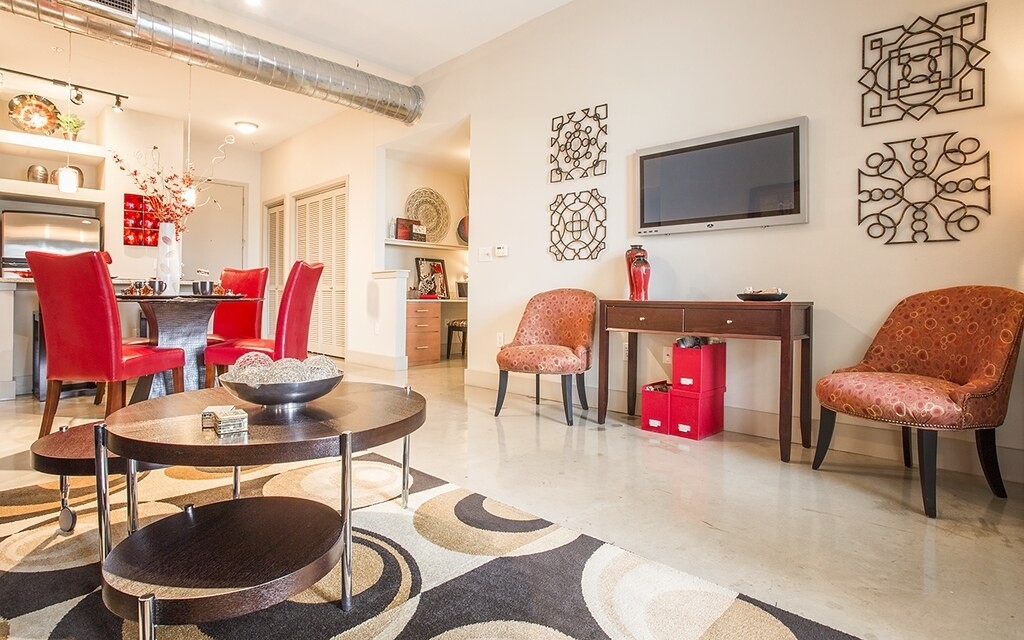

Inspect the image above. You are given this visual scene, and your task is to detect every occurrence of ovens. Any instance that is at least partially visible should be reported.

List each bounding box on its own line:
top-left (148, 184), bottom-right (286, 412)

top-left (2, 210), bottom-right (101, 268)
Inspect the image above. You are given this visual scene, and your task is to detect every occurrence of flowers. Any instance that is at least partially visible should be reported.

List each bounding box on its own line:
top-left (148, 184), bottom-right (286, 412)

top-left (109, 135), bottom-right (236, 243)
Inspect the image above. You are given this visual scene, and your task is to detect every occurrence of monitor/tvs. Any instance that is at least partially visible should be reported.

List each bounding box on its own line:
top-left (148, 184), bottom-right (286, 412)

top-left (635, 115), bottom-right (809, 235)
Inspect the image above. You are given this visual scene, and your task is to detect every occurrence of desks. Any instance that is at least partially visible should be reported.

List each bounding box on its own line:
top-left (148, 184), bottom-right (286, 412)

top-left (114, 295), bottom-right (266, 400)
top-left (29, 382), bottom-right (428, 640)
top-left (595, 300), bottom-right (813, 461)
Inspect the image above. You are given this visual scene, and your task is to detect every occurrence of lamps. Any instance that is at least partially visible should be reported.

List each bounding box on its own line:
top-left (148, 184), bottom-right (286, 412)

top-left (0, 68), bottom-right (129, 113)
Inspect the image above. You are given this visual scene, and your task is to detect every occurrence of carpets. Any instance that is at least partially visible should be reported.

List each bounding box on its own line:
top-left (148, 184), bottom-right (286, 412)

top-left (0, 450), bottom-right (862, 640)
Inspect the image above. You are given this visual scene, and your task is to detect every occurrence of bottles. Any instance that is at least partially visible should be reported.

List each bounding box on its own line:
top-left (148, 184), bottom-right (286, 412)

top-left (631, 254), bottom-right (650, 302)
top-left (625, 245), bottom-right (647, 301)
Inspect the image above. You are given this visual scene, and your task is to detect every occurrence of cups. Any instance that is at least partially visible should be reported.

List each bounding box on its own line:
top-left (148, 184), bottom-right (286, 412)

top-left (193, 282), bottom-right (202, 295)
top-left (200, 281), bottom-right (214, 295)
top-left (148, 280), bottom-right (167, 296)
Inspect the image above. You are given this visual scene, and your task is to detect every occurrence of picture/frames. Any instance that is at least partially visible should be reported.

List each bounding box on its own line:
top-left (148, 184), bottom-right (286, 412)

top-left (416, 258), bottom-right (450, 299)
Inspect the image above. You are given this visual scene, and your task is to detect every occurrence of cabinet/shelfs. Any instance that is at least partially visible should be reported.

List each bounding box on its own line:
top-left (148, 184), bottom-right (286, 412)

top-left (385, 239), bottom-right (469, 302)
top-left (405, 300), bottom-right (441, 368)
top-left (0, 130), bottom-right (112, 286)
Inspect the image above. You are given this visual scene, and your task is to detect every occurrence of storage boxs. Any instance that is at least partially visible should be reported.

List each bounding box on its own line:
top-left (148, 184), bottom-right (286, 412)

top-left (641, 335), bottom-right (727, 440)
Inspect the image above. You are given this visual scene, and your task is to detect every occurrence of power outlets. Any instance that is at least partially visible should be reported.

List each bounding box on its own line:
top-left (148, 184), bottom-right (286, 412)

top-left (623, 343), bottom-right (629, 361)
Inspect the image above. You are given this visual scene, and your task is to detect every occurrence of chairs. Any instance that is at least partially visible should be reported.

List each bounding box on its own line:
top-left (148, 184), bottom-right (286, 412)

top-left (495, 288), bottom-right (597, 426)
top-left (812, 285), bottom-right (1024, 518)
top-left (25, 251), bottom-right (324, 439)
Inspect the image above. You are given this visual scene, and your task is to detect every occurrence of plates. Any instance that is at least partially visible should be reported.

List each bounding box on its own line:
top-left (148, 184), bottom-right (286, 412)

top-left (7, 93), bottom-right (62, 137)
top-left (737, 294), bottom-right (788, 302)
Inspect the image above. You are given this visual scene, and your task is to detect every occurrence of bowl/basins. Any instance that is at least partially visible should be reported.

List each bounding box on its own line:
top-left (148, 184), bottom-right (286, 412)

top-left (219, 370), bottom-right (344, 412)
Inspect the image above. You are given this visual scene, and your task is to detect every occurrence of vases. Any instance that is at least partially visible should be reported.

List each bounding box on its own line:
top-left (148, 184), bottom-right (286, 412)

top-left (631, 254), bottom-right (651, 300)
top-left (156, 223), bottom-right (180, 295)
top-left (626, 244), bottom-right (647, 299)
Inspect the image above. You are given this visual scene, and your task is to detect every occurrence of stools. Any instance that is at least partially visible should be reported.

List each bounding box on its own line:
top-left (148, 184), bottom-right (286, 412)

top-left (447, 319), bottom-right (468, 360)
